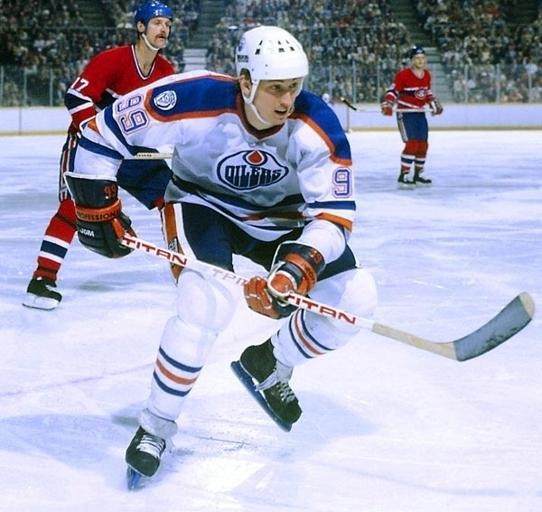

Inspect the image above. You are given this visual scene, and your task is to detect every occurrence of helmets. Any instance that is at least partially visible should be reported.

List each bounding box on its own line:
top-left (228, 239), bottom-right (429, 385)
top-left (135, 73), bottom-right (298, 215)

top-left (236, 26), bottom-right (308, 104)
top-left (135, 0), bottom-right (173, 25)
top-left (410, 47), bottom-right (426, 59)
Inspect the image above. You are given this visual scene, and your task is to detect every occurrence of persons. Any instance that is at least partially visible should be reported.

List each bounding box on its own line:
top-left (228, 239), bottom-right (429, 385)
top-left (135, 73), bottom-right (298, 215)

top-left (380, 45), bottom-right (444, 185)
top-left (61, 25), bottom-right (378, 479)
top-left (25, 0), bottom-right (179, 302)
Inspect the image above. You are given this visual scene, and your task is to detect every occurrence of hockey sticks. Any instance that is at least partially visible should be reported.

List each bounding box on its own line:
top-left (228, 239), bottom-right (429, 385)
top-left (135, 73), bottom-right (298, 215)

top-left (116, 229), bottom-right (535, 362)
top-left (339, 96), bottom-right (436, 112)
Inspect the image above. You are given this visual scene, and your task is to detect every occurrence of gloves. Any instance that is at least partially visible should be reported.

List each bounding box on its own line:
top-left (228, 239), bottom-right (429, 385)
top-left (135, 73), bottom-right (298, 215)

top-left (244, 240), bottom-right (326, 319)
top-left (380, 99), bottom-right (392, 115)
top-left (62, 171), bottom-right (136, 259)
top-left (429, 98), bottom-right (444, 115)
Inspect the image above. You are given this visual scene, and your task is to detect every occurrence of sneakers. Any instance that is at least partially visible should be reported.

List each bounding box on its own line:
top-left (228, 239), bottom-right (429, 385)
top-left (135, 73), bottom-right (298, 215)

top-left (126, 424), bottom-right (167, 478)
top-left (398, 172), bottom-right (414, 183)
top-left (240, 330), bottom-right (302, 424)
top-left (416, 171), bottom-right (431, 185)
top-left (27, 274), bottom-right (61, 302)
